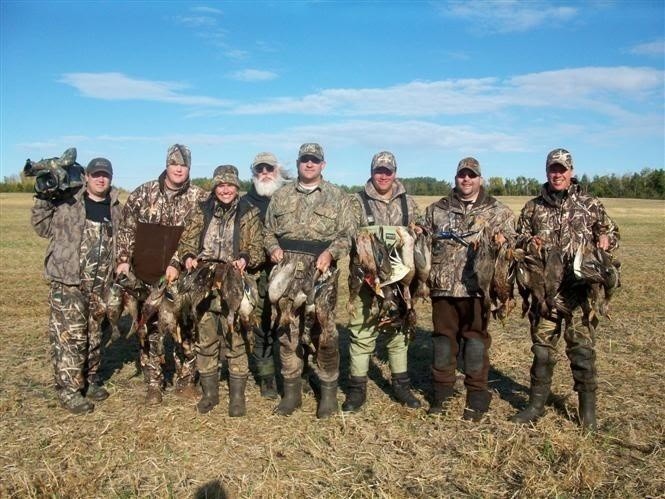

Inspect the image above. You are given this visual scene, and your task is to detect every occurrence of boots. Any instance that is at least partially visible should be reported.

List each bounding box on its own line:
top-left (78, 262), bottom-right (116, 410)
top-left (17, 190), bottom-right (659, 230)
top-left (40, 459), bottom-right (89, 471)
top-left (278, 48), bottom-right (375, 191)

top-left (392, 371), bottom-right (486, 420)
top-left (579, 391), bottom-right (597, 436)
top-left (278, 376), bottom-right (367, 417)
top-left (197, 372), bottom-right (246, 416)
top-left (509, 383), bottom-right (549, 423)
top-left (144, 365), bottom-right (162, 407)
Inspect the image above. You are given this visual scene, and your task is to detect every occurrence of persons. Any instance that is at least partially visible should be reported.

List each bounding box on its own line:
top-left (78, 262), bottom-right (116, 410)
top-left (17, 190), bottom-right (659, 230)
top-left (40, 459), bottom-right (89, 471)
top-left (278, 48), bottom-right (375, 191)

top-left (510, 149), bottom-right (621, 439)
top-left (178, 165), bottom-right (266, 417)
top-left (342, 151), bottom-right (424, 411)
top-left (421, 157), bottom-right (516, 422)
top-left (240, 154), bottom-right (286, 399)
top-left (116, 144), bottom-right (209, 405)
top-left (31, 158), bottom-right (124, 415)
top-left (263, 143), bottom-right (356, 418)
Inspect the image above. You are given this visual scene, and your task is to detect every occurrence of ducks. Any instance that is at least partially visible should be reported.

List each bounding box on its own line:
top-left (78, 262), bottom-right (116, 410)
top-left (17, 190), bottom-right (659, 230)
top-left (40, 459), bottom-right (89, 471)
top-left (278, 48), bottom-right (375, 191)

top-left (105, 259), bottom-right (340, 354)
top-left (355, 227), bottom-right (431, 334)
top-left (473, 227), bottom-right (619, 328)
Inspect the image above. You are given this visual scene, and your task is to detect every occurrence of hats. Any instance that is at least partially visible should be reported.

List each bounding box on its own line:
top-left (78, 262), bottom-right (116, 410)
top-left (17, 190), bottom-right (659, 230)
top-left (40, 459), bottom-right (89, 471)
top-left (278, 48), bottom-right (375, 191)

top-left (547, 149), bottom-right (572, 170)
top-left (457, 158), bottom-right (481, 175)
top-left (167, 144), bottom-right (191, 167)
top-left (211, 152), bottom-right (276, 188)
top-left (372, 152), bottom-right (396, 172)
top-left (299, 143), bottom-right (324, 161)
top-left (87, 158), bottom-right (112, 176)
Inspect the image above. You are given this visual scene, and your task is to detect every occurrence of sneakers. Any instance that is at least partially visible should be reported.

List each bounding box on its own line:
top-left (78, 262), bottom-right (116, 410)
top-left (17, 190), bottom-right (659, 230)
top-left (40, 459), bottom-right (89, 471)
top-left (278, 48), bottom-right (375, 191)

top-left (59, 387), bottom-right (109, 412)
top-left (261, 375), bottom-right (277, 399)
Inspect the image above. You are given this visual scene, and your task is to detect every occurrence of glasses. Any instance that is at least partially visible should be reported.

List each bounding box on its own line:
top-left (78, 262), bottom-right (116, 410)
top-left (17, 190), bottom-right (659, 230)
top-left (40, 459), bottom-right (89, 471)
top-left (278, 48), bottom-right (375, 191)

top-left (458, 171), bottom-right (476, 177)
top-left (301, 155), bottom-right (320, 164)
top-left (255, 166), bottom-right (274, 172)
